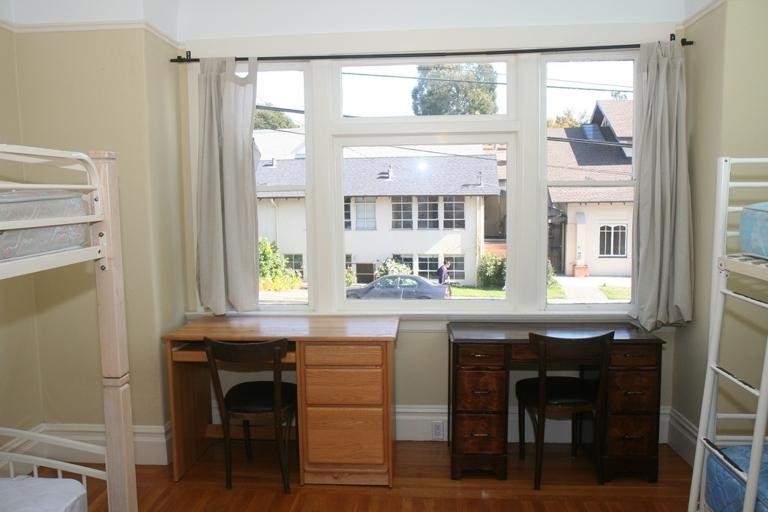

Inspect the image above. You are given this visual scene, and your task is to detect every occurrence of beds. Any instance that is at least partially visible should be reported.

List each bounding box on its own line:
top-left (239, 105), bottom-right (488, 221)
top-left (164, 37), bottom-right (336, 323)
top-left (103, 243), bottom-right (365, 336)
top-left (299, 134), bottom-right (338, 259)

top-left (688, 158), bottom-right (768, 511)
top-left (0, 146), bottom-right (138, 511)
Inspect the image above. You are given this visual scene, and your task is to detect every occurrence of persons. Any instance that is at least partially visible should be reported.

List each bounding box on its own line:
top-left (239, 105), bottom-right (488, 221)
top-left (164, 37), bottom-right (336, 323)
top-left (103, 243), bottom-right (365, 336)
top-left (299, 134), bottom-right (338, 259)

top-left (437, 259), bottom-right (453, 298)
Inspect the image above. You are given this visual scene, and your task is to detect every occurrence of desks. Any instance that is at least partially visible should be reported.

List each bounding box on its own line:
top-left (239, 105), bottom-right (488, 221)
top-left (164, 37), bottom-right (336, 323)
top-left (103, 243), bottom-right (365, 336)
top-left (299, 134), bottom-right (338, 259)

top-left (448, 324), bottom-right (666, 483)
top-left (162, 318), bottom-right (399, 489)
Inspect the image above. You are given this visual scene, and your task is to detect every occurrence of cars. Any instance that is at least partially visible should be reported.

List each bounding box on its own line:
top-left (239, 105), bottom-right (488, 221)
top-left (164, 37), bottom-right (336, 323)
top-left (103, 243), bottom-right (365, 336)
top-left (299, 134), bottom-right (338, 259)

top-left (345, 276), bottom-right (450, 300)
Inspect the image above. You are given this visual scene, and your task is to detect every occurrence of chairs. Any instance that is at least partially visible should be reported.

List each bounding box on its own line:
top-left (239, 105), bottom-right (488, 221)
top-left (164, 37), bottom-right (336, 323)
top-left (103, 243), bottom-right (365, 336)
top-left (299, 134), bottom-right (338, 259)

top-left (515, 331), bottom-right (615, 489)
top-left (204, 337), bottom-right (296, 493)
